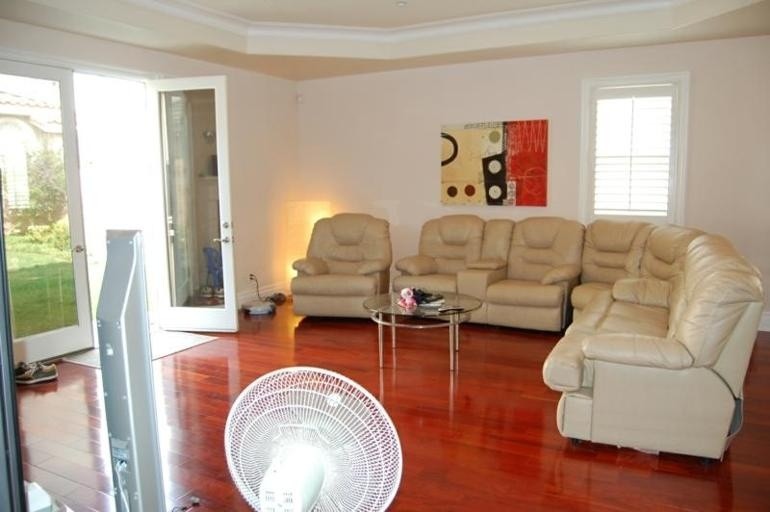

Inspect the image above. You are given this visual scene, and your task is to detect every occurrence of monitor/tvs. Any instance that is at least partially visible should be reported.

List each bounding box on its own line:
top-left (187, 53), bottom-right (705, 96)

top-left (0, 174), bottom-right (27, 512)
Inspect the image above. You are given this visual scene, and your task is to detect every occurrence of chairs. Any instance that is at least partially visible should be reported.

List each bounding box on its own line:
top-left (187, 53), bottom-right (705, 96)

top-left (288, 212), bottom-right (394, 321)
top-left (635, 222), bottom-right (705, 281)
top-left (394, 212), bottom-right (488, 326)
top-left (569, 219), bottom-right (661, 326)
top-left (481, 215), bottom-right (586, 335)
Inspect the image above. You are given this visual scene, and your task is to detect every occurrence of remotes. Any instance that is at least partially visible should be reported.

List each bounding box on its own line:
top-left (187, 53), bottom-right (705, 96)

top-left (437, 306), bottom-right (465, 312)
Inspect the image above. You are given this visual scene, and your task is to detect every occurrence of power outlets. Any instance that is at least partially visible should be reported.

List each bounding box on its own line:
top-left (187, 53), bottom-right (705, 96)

top-left (248, 273), bottom-right (254, 283)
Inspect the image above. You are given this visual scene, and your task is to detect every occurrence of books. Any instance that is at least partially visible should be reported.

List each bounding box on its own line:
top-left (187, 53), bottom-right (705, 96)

top-left (424, 294), bottom-right (445, 304)
top-left (417, 300), bottom-right (442, 307)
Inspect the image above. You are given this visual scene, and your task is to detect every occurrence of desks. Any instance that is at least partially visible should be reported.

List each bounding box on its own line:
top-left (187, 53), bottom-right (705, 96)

top-left (356, 286), bottom-right (484, 420)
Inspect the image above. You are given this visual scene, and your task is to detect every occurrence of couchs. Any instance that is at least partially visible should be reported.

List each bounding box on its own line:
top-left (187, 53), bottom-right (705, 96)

top-left (541, 232), bottom-right (767, 466)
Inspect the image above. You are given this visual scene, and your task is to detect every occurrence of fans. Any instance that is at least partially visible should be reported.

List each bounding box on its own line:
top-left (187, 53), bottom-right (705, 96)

top-left (223, 364), bottom-right (405, 512)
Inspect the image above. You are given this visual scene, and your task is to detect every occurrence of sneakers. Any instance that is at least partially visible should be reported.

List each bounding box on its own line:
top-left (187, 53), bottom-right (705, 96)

top-left (15, 362), bottom-right (58, 384)
top-left (15, 362), bottom-right (46, 375)
top-left (200, 286), bottom-right (213, 297)
top-left (214, 288), bottom-right (224, 298)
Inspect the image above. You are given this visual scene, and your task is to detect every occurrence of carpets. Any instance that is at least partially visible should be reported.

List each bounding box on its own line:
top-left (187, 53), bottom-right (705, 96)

top-left (62, 330), bottom-right (221, 370)
